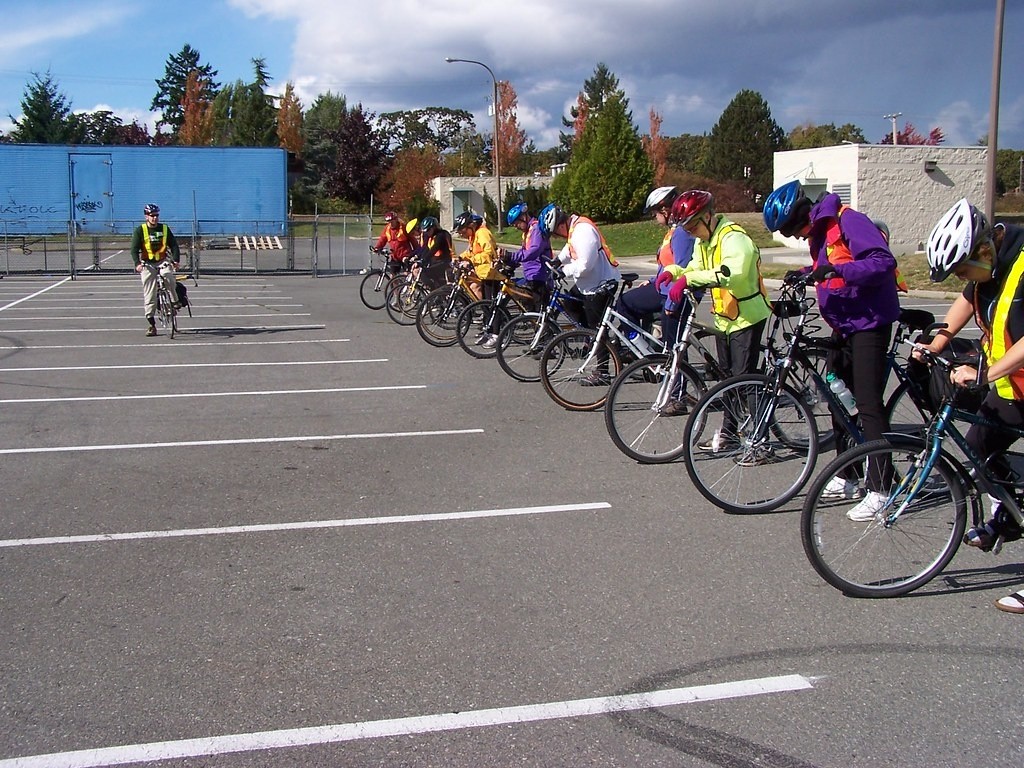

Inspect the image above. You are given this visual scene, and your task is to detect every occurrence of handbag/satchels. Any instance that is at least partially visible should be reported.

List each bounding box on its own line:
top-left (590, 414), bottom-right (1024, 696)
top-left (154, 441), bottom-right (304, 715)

top-left (906, 333), bottom-right (988, 416)
top-left (156, 281), bottom-right (188, 308)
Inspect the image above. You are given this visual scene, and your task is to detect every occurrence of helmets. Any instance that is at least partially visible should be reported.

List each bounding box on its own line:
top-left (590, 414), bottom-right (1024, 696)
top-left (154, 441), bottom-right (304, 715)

top-left (537, 203), bottom-right (565, 238)
top-left (642, 186), bottom-right (677, 215)
top-left (763, 180), bottom-right (806, 233)
top-left (406, 219), bottom-right (418, 234)
top-left (670, 188), bottom-right (712, 227)
top-left (507, 205), bottom-right (527, 224)
top-left (384, 211), bottom-right (399, 221)
top-left (927, 199), bottom-right (990, 283)
top-left (452, 212), bottom-right (473, 233)
top-left (143, 204), bottom-right (160, 216)
top-left (420, 218), bottom-right (436, 231)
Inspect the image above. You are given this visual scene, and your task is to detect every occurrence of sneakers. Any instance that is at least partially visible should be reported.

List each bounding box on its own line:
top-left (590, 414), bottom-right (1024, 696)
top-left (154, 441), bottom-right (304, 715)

top-left (697, 434), bottom-right (741, 451)
top-left (482, 335), bottom-right (503, 349)
top-left (733, 448), bottom-right (775, 467)
top-left (659, 402), bottom-right (687, 417)
top-left (819, 475), bottom-right (861, 501)
top-left (531, 349), bottom-right (555, 360)
top-left (847, 488), bottom-right (893, 521)
top-left (522, 346), bottom-right (542, 355)
top-left (577, 371), bottom-right (610, 387)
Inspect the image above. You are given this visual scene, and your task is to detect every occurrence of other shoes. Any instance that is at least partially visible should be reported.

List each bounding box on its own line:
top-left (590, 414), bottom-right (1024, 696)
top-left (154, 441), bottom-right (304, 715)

top-left (145, 326), bottom-right (157, 336)
top-left (390, 300), bottom-right (405, 308)
top-left (173, 302), bottom-right (181, 308)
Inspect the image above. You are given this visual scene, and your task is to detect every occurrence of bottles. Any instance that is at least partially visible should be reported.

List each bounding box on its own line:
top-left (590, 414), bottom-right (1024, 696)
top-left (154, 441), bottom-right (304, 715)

top-left (629, 331), bottom-right (658, 356)
top-left (826, 370), bottom-right (860, 416)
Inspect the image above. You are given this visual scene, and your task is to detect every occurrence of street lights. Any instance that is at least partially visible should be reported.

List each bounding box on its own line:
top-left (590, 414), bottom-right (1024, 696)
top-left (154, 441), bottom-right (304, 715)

top-left (883, 111), bottom-right (902, 148)
top-left (445, 57), bottom-right (506, 236)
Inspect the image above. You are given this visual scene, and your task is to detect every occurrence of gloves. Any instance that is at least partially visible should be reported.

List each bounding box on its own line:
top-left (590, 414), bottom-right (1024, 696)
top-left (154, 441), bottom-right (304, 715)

top-left (813, 265), bottom-right (837, 282)
top-left (656, 271), bottom-right (672, 292)
top-left (785, 270), bottom-right (802, 284)
top-left (669, 275), bottom-right (687, 303)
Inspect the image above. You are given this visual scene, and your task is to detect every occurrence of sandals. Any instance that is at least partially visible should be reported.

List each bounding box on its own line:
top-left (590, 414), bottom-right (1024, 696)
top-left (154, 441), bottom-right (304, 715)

top-left (962, 514), bottom-right (1021, 546)
top-left (995, 581), bottom-right (1024, 615)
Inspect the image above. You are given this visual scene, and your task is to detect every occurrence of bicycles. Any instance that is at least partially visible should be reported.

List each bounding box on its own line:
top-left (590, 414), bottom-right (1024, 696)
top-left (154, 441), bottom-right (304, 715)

top-left (799, 322), bottom-right (1024, 600)
top-left (139, 260), bottom-right (182, 340)
top-left (358, 244), bottom-right (977, 516)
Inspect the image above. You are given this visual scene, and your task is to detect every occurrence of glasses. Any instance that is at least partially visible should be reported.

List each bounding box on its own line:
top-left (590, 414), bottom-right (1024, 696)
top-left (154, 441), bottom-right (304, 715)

top-left (684, 215), bottom-right (703, 236)
top-left (150, 214), bottom-right (159, 218)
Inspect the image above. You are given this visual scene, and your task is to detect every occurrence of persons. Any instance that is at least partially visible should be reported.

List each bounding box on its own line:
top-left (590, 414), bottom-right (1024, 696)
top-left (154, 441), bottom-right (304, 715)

top-left (656, 190), bottom-right (776, 466)
top-left (131, 204), bottom-right (183, 336)
top-left (763, 179), bottom-right (901, 522)
top-left (538, 204), bottom-right (620, 385)
top-left (373, 212), bottom-right (413, 307)
top-left (452, 211), bottom-right (504, 348)
top-left (403, 218), bottom-right (457, 322)
top-left (491, 204), bottom-right (556, 360)
top-left (912, 199), bottom-right (1024, 613)
top-left (646, 187), bottom-right (707, 416)
